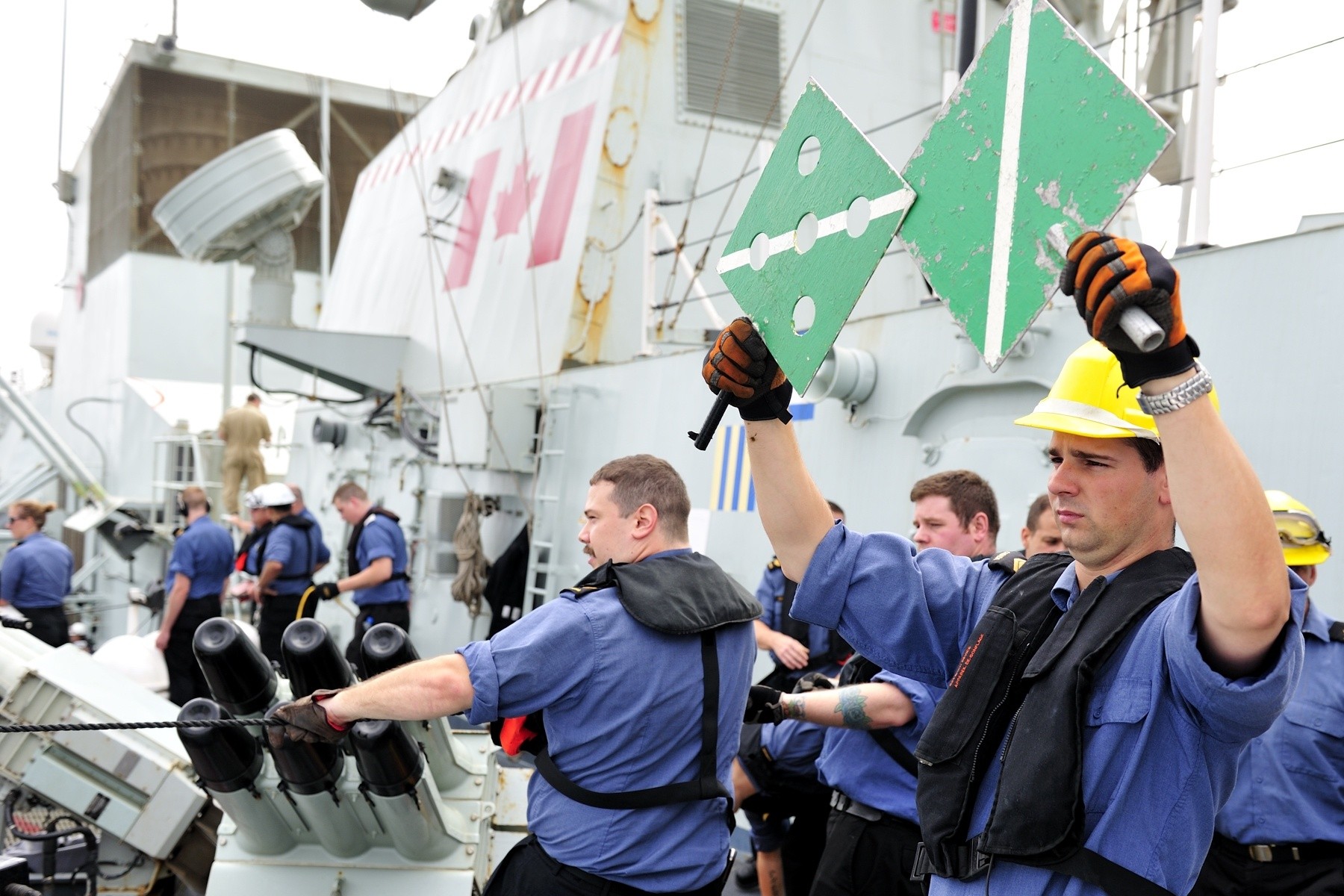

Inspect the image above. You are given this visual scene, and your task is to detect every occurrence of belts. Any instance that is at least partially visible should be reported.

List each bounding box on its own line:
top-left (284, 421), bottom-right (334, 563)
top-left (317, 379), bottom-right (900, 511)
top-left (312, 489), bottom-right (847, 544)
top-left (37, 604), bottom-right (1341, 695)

top-left (557, 859), bottom-right (645, 896)
top-left (1211, 831), bottom-right (1343, 867)
top-left (829, 790), bottom-right (914, 825)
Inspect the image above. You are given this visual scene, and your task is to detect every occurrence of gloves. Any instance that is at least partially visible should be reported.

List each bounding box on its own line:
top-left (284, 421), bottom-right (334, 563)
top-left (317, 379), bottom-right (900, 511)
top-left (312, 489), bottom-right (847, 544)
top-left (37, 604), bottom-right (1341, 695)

top-left (743, 686), bottom-right (788, 727)
top-left (317, 583), bottom-right (339, 600)
top-left (267, 689), bottom-right (358, 750)
top-left (701, 316), bottom-right (794, 425)
top-left (1058, 230), bottom-right (1201, 399)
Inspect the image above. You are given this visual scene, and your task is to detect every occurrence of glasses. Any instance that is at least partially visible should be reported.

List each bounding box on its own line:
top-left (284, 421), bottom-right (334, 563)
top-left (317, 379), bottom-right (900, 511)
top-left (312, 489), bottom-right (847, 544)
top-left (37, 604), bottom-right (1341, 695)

top-left (9, 516), bottom-right (26, 525)
top-left (1269, 510), bottom-right (1331, 553)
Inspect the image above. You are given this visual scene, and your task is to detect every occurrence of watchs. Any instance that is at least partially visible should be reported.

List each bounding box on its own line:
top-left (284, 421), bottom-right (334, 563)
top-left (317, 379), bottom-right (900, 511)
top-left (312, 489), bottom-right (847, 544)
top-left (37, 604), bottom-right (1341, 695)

top-left (1136, 361), bottom-right (1212, 415)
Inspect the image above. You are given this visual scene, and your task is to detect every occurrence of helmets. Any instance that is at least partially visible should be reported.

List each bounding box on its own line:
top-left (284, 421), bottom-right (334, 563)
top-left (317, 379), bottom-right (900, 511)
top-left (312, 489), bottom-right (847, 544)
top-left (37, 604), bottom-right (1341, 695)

top-left (262, 482), bottom-right (296, 506)
top-left (1012, 338), bottom-right (1223, 446)
top-left (246, 485), bottom-right (269, 509)
top-left (1260, 489), bottom-right (1331, 567)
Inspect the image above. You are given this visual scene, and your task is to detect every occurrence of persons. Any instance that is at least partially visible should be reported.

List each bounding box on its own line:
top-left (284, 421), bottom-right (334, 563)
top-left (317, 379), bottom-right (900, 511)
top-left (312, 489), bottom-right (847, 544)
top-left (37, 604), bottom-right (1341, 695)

top-left (67, 621), bottom-right (91, 655)
top-left (155, 486), bottom-right (236, 708)
top-left (997, 494), bottom-right (1067, 572)
top-left (239, 486), bottom-right (274, 614)
top-left (741, 470), bottom-right (1001, 896)
top-left (263, 453), bottom-right (758, 896)
top-left (755, 499), bottom-right (845, 669)
top-left (700, 231), bottom-right (1311, 896)
top-left (1180, 486), bottom-right (1344, 893)
top-left (316, 482), bottom-right (412, 681)
top-left (0, 499), bottom-right (74, 649)
top-left (246, 482), bottom-right (321, 679)
top-left (288, 485), bottom-right (323, 542)
